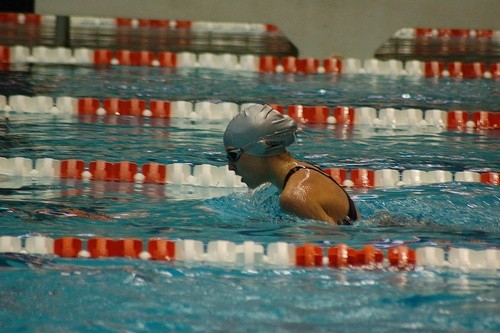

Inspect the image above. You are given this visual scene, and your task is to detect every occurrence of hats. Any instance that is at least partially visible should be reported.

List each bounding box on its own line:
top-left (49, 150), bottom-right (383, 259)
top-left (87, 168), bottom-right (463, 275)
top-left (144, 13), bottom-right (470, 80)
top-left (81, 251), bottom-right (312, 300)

top-left (224, 104), bottom-right (297, 155)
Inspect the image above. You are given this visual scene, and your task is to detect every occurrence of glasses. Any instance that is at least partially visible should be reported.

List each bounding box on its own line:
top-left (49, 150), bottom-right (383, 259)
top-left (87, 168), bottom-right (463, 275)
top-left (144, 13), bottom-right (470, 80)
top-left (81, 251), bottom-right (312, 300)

top-left (226, 129), bottom-right (296, 160)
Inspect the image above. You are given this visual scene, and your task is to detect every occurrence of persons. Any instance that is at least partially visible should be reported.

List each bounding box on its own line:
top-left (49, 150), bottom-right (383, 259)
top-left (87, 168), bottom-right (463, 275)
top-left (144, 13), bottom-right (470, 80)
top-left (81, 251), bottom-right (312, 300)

top-left (223, 104), bottom-right (363, 226)
